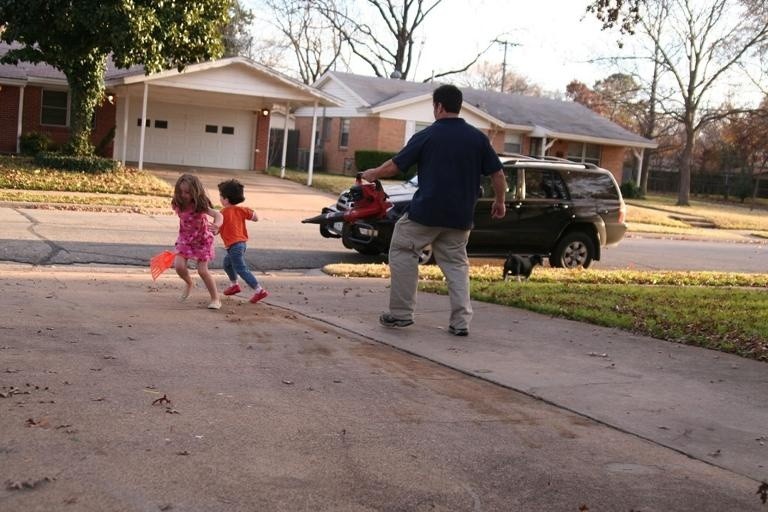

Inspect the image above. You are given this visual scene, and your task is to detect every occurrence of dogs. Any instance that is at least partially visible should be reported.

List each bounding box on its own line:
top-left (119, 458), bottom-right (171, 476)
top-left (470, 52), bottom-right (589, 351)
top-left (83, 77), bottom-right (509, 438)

top-left (500, 250), bottom-right (544, 284)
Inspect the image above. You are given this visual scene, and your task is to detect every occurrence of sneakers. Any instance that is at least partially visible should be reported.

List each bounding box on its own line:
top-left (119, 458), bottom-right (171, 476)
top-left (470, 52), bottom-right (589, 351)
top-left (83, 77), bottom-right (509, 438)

top-left (379, 312), bottom-right (416, 327)
top-left (222, 284), bottom-right (243, 295)
top-left (207, 297), bottom-right (222, 311)
top-left (447, 324), bottom-right (469, 336)
top-left (249, 288), bottom-right (270, 304)
top-left (176, 292), bottom-right (189, 303)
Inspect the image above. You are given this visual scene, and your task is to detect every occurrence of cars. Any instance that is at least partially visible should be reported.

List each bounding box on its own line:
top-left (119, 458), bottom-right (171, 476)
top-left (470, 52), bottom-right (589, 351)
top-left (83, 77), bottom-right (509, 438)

top-left (320, 152), bottom-right (628, 269)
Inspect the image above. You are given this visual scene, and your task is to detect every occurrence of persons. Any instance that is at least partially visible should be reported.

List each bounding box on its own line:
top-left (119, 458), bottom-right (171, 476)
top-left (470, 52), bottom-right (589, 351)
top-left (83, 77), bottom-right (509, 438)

top-left (210, 179), bottom-right (269, 304)
top-left (171, 173), bottom-right (223, 311)
top-left (360, 84), bottom-right (508, 337)
